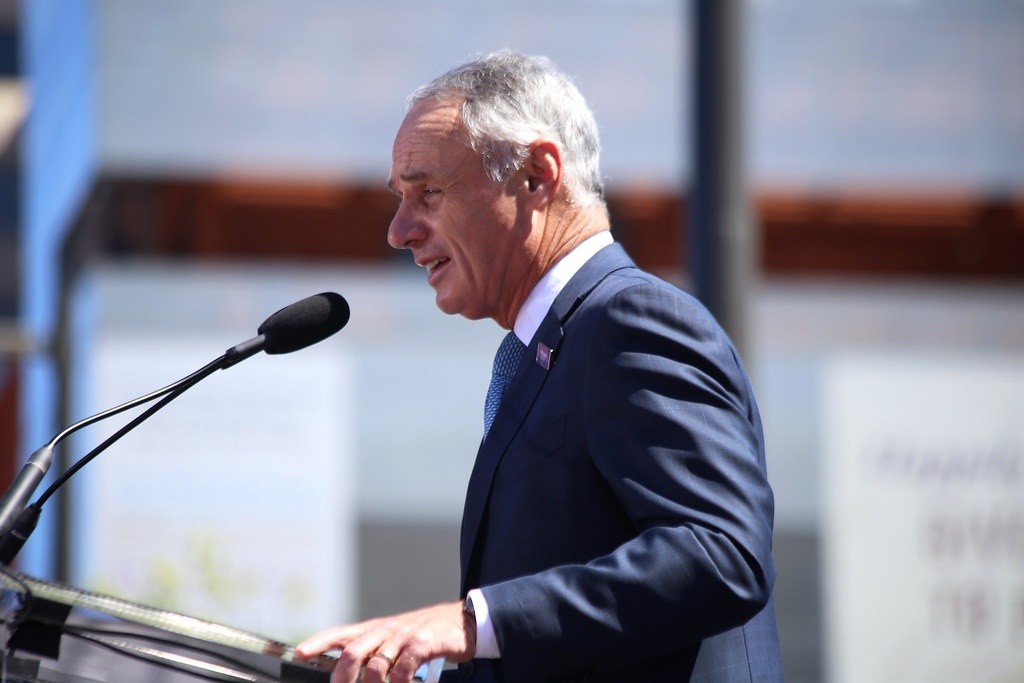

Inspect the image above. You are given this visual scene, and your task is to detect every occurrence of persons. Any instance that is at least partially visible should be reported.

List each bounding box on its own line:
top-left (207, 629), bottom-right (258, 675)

top-left (294, 50), bottom-right (783, 683)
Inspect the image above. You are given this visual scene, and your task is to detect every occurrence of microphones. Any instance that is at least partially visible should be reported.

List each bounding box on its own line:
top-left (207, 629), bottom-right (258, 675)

top-left (0, 292), bottom-right (350, 568)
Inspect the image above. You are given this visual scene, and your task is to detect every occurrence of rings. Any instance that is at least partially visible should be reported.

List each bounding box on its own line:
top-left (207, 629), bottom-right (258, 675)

top-left (374, 652), bottom-right (394, 665)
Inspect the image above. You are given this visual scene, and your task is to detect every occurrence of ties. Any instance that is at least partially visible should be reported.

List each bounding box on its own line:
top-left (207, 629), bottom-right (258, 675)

top-left (482, 330), bottom-right (530, 443)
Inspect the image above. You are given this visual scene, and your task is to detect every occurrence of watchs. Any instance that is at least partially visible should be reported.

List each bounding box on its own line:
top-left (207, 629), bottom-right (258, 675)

top-left (463, 597), bottom-right (476, 617)
